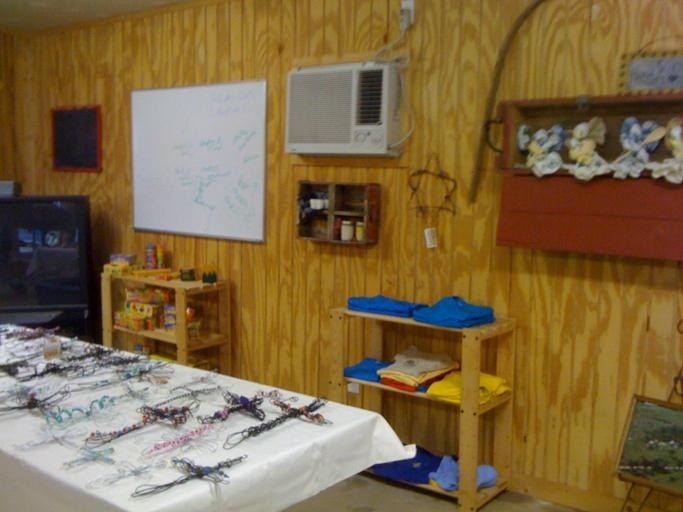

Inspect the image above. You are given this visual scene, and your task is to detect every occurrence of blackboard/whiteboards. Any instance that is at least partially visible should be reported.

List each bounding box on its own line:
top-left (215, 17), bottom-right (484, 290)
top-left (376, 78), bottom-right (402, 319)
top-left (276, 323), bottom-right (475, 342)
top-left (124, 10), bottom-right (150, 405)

top-left (132, 81), bottom-right (266, 243)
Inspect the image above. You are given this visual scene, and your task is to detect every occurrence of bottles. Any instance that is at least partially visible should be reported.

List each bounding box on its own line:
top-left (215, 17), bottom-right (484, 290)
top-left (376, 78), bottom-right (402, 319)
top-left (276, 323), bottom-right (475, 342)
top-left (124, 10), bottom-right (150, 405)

top-left (144, 242), bottom-right (165, 270)
top-left (340, 221), bottom-right (364, 243)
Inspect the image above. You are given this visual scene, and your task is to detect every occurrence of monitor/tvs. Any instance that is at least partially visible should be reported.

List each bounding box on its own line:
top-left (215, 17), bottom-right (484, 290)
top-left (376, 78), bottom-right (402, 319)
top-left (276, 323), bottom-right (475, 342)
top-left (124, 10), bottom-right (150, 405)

top-left (0, 193), bottom-right (95, 313)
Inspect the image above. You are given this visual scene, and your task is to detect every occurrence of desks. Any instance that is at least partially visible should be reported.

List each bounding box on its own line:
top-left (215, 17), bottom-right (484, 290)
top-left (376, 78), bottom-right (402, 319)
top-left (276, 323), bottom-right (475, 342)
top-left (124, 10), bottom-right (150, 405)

top-left (1, 317), bottom-right (417, 511)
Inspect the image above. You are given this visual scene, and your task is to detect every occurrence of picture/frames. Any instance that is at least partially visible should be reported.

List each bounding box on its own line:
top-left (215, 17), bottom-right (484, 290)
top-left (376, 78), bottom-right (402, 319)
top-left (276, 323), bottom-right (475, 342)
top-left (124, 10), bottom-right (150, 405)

top-left (611, 393), bottom-right (683, 498)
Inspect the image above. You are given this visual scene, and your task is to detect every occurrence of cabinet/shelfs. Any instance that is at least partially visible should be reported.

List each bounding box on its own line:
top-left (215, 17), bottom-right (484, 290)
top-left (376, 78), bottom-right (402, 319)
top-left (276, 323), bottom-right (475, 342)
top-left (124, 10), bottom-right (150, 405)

top-left (97, 264), bottom-right (232, 371)
top-left (295, 177), bottom-right (381, 249)
top-left (331, 291), bottom-right (508, 506)
top-left (495, 93), bottom-right (683, 262)
